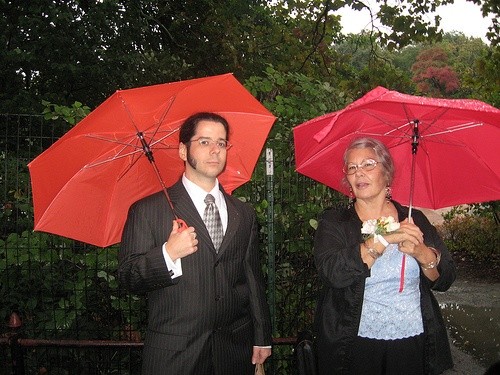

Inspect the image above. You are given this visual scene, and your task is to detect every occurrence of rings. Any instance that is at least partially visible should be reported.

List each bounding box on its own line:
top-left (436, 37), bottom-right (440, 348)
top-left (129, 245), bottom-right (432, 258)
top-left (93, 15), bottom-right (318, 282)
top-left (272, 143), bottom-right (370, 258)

top-left (191, 233), bottom-right (195, 238)
top-left (402, 242), bottom-right (405, 247)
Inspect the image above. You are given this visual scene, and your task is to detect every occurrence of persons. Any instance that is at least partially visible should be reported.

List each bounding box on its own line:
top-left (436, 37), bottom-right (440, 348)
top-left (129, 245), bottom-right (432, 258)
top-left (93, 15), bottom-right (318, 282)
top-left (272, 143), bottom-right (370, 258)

top-left (314, 138), bottom-right (456, 375)
top-left (118, 112), bottom-right (273, 375)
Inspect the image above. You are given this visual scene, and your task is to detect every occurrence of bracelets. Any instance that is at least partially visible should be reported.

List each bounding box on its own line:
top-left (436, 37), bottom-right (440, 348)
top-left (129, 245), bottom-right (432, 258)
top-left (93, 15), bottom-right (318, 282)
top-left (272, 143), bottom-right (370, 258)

top-left (418, 246), bottom-right (441, 270)
top-left (364, 240), bottom-right (383, 259)
top-left (361, 216), bottom-right (400, 248)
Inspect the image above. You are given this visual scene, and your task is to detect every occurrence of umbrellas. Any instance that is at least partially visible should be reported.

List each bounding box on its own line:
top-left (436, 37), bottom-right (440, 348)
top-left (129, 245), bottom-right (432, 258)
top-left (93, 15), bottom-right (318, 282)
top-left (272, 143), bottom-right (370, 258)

top-left (292, 86), bottom-right (500, 292)
top-left (27, 72), bottom-right (275, 249)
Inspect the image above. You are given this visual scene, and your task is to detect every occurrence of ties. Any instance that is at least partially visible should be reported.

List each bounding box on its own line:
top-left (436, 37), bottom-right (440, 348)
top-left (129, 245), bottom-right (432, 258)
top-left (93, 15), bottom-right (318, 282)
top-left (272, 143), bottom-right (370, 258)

top-left (203, 193), bottom-right (224, 254)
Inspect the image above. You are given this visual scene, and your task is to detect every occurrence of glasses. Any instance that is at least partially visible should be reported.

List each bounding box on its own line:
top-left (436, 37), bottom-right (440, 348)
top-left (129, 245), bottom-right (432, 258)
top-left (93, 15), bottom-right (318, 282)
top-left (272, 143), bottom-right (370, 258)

top-left (342, 158), bottom-right (380, 175)
top-left (189, 137), bottom-right (232, 151)
